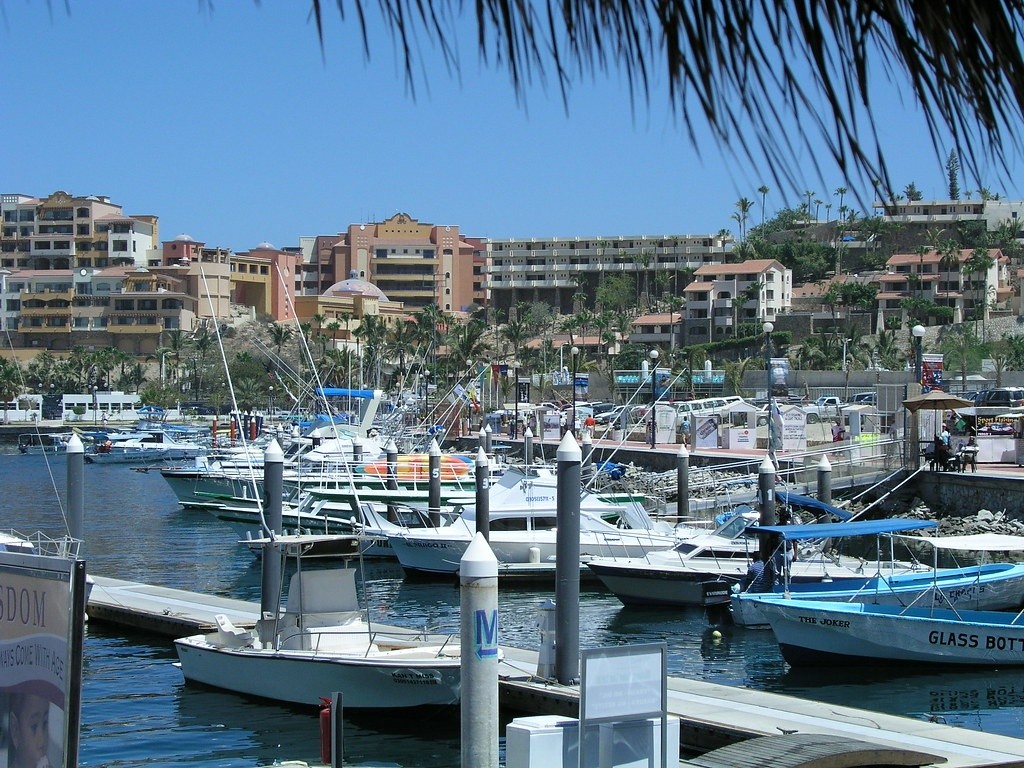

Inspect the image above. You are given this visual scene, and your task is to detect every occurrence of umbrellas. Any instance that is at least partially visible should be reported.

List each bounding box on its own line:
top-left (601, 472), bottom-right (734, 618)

top-left (902, 390), bottom-right (974, 471)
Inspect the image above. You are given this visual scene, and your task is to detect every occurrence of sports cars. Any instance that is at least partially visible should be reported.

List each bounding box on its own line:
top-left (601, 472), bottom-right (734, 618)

top-left (986, 423), bottom-right (1014, 435)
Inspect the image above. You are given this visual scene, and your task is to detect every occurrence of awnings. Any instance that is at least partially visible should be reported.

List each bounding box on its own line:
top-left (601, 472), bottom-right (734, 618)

top-left (996, 413), bottom-right (1024, 438)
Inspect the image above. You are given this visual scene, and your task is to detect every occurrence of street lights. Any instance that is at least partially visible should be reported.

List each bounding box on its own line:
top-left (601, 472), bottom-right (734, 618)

top-left (762, 321), bottom-right (774, 451)
top-left (571, 347), bottom-right (579, 437)
top-left (38, 383), bottom-right (42, 392)
top-left (912, 324), bottom-right (926, 383)
top-left (93, 386), bottom-right (99, 425)
top-left (650, 350), bottom-right (659, 448)
top-left (466, 359), bottom-right (473, 435)
top-left (513, 360), bottom-right (520, 439)
top-left (560, 342), bottom-right (568, 374)
top-left (103, 383), bottom-right (107, 393)
top-left (843, 338), bottom-right (851, 365)
top-left (49, 384), bottom-right (55, 396)
top-left (269, 386), bottom-right (273, 425)
top-left (425, 370), bottom-right (430, 431)
top-left (162, 352), bottom-right (171, 391)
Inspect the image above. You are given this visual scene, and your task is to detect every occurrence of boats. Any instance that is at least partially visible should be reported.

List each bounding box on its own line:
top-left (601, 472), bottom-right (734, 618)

top-left (170, 528), bottom-right (505, 723)
top-left (729, 563), bottom-right (1024, 631)
top-left (18, 259), bottom-right (677, 582)
top-left (748, 597), bottom-right (1024, 681)
top-left (586, 512), bottom-right (935, 612)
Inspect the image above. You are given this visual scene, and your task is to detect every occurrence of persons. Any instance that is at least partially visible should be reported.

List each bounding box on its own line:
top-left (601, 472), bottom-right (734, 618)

top-left (680, 416), bottom-right (692, 447)
top-left (94, 438), bottom-right (112, 454)
top-left (646, 416), bottom-right (657, 446)
top-left (8, 693), bottom-right (49, 768)
top-left (522, 413), bottom-right (535, 436)
top-left (831, 421), bottom-right (845, 457)
top-left (925, 423), bottom-right (980, 472)
top-left (509, 416), bottom-right (514, 440)
top-left (583, 414), bottom-right (594, 439)
top-left (602, 421), bottom-right (620, 439)
top-left (560, 413), bottom-right (568, 438)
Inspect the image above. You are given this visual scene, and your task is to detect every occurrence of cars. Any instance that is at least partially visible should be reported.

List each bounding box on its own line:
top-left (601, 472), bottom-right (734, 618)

top-left (479, 392), bottom-right (877, 433)
top-left (948, 386), bottom-right (1024, 407)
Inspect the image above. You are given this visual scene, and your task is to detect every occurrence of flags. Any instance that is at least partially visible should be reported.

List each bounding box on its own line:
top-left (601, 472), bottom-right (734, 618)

top-left (262, 362), bottom-right (274, 380)
top-left (430, 425), bottom-right (443, 435)
top-left (454, 384), bottom-right (480, 413)
